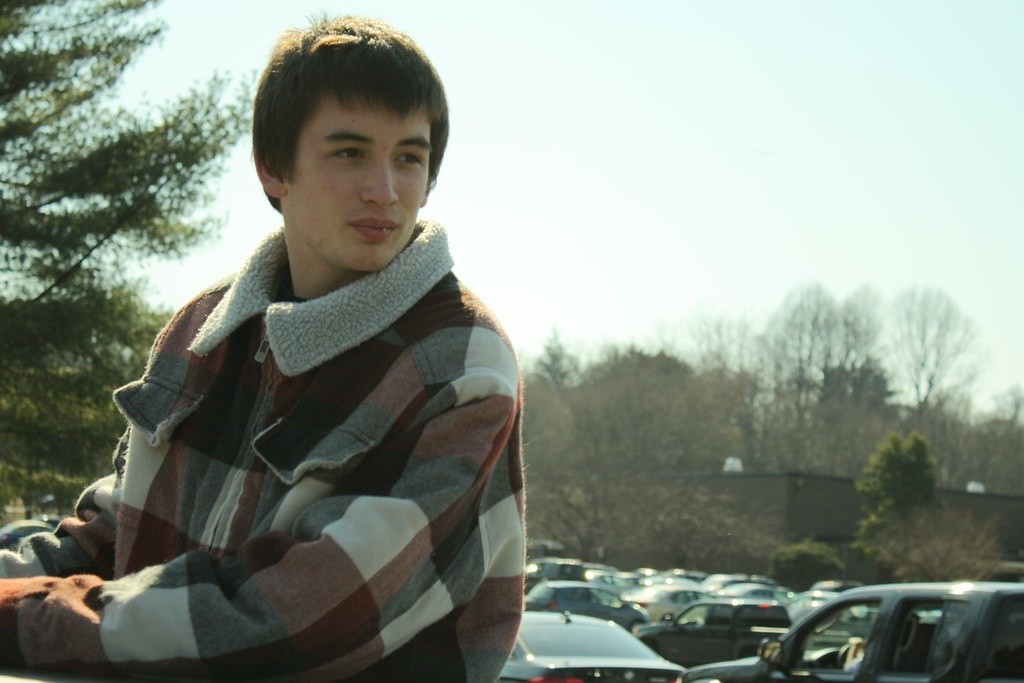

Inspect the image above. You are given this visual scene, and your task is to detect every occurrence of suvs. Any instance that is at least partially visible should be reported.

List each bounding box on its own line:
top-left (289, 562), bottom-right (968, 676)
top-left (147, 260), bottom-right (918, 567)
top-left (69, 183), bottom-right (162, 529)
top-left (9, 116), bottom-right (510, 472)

top-left (676, 579), bottom-right (1023, 682)
top-left (631, 597), bottom-right (795, 672)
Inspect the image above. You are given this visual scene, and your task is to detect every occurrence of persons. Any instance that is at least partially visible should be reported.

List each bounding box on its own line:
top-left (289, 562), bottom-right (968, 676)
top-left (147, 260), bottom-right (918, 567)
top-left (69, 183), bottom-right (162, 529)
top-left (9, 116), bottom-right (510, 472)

top-left (844, 636), bottom-right (867, 673)
top-left (0, 16), bottom-right (527, 683)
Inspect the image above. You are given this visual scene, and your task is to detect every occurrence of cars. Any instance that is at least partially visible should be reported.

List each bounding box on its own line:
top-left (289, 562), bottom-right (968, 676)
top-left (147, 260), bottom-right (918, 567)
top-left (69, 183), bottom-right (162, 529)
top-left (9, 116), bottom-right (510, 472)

top-left (0, 518), bottom-right (57, 550)
top-left (496, 609), bottom-right (688, 683)
top-left (498, 540), bottom-right (943, 628)
